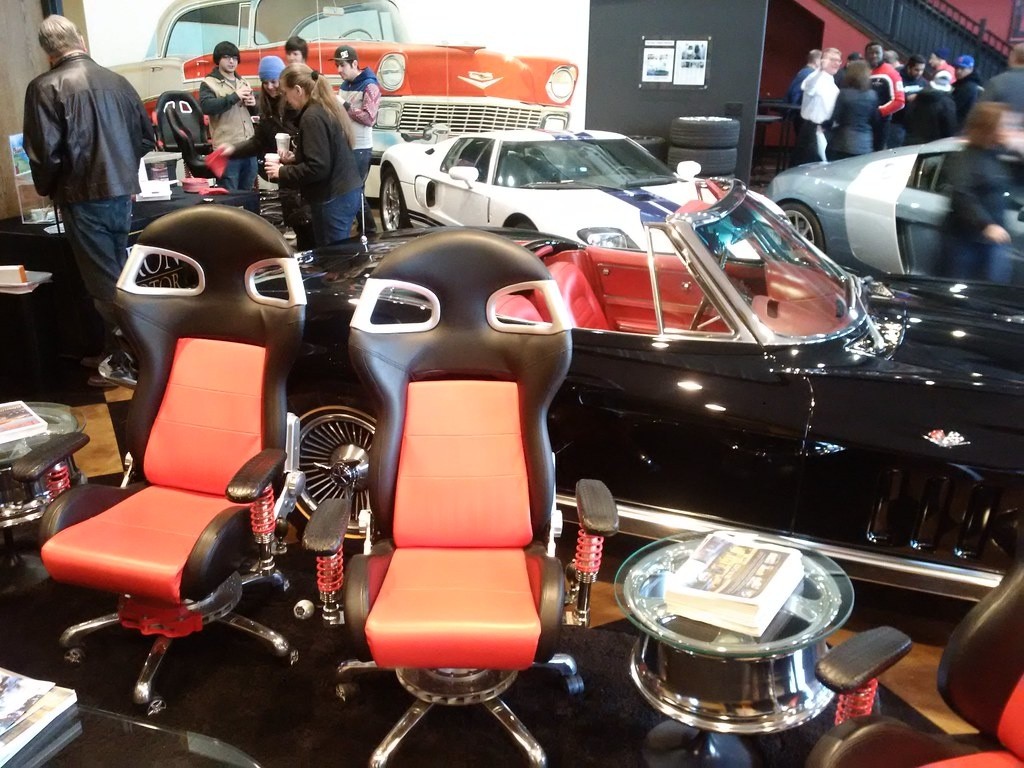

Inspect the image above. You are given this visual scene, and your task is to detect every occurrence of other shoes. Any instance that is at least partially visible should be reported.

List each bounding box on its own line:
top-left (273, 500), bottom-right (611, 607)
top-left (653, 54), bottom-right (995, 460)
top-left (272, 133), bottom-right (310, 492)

top-left (82, 349), bottom-right (110, 369)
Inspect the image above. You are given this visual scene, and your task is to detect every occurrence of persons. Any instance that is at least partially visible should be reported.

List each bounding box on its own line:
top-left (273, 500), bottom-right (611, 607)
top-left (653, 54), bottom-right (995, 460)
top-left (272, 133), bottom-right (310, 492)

top-left (942, 44), bottom-right (1024, 285)
top-left (22, 14), bottom-right (157, 370)
top-left (198, 41), bottom-right (258, 190)
top-left (328, 45), bottom-right (381, 238)
top-left (213, 36), bottom-right (323, 251)
top-left (786, 41), bottom-right (984, 165)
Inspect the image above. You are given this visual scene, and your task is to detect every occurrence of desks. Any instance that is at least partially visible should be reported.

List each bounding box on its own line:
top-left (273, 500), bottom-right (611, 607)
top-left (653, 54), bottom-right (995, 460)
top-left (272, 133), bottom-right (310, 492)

top-left (755, 97), bottom-right (801, 175)
top-left (0, 402), bottom-right (86, 599)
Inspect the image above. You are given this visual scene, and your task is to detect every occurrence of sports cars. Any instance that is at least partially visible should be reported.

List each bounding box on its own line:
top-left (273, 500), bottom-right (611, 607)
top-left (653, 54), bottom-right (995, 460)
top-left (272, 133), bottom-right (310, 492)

top-left (286, 175), bottom-right (1024, 557)
top-left (380, 128), bottom-right (793, 257)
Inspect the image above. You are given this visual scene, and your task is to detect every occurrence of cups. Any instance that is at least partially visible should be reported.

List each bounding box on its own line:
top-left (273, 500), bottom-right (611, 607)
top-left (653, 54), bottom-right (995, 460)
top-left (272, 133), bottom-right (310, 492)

top-left (265, 153), bottom-right (280, 164)
top-left (275, 133), bottom-right (291, 156)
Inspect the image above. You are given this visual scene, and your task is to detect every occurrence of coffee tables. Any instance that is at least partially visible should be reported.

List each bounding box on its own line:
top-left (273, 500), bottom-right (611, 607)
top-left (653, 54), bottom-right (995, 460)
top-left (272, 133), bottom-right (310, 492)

top-left (613, 529), bottom-right (855, 734)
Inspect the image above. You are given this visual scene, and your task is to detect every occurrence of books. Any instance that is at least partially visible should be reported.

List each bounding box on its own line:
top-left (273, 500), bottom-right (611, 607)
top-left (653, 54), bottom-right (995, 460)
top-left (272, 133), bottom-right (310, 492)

top-left (0, 666), bottom-right (78, 768)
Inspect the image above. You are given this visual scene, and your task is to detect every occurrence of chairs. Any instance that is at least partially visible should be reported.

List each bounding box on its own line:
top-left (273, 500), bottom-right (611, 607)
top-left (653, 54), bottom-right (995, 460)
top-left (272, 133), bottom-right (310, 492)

top-left (151, 91), bottom-right (217, 188)
top-left (38, 203), bottom-right (313, 715)
top-left (302, 226), bottom-right (619, 768)
top-left (804, 563), bottom-right (1024, 768)
top-left (495, 259), bottom-right (611, 331)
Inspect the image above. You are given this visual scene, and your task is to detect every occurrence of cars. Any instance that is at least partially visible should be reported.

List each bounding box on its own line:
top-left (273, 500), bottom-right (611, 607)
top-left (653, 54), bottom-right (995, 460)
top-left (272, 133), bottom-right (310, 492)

top-left (89, 0), bottom-right (582, 154)
top-left (762, 132), bottom-right (1024, 289)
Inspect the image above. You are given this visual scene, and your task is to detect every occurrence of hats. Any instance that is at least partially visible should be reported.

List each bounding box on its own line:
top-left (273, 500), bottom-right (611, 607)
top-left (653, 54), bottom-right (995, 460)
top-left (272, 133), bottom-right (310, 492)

top-left (258, 55), bottom-right (285, 81)
top-left (932, 47), bottom-right (951, 58)
top-left (328, 45), bottom-right (358, 61)
top-left (930, 70), bottom-right (952, 92)
top-left (955, 55), bottom-right (975, 68)
